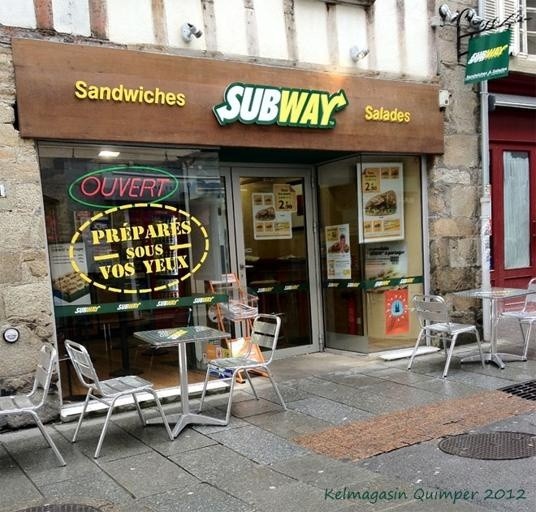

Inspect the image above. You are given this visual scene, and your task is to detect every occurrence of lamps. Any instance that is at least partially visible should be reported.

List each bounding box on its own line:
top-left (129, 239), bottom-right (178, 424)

top-left (180, 23), bottom-right (203, 42)
top-left (350, 46), bottom-right (370, 62)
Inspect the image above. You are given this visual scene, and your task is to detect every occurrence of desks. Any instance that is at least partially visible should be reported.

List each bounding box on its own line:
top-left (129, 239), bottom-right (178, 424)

top-left (133, 326), bottom-right (231, 438)
top-left (456, 286), bottom-right (536, 369)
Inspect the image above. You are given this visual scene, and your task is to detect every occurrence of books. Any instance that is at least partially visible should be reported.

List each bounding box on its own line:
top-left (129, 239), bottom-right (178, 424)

top-left (218, 298), bottom-right (258, 323)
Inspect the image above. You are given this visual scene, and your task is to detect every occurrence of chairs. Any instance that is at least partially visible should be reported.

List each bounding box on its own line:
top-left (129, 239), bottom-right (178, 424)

top-left (489, 277), bottom-right (536, 360)
top-left (198, 314), bottom-right (287, 423)
top-left (64, 339), bottom-right (174, 459)
top-left (0, 341), bottom-right (67, 466)
top-left (408, 294), bottom-right (485, 377)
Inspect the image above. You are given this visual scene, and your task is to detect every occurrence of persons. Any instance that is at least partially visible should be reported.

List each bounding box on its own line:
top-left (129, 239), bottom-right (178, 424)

top-left (333, 232), bottom-right (348, 253)
